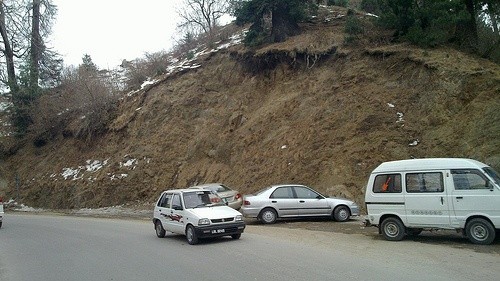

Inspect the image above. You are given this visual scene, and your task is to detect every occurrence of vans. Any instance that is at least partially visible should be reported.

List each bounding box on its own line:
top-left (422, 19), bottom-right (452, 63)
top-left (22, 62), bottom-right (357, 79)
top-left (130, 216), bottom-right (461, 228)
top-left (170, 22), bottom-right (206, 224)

top-left (361, 157), bottom-right (500, 245)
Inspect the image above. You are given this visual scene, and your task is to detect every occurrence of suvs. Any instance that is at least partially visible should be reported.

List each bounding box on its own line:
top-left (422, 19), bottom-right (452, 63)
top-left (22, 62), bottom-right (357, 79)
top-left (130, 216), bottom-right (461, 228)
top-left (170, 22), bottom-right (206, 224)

top-left (152, 186), bottom-right (246, 245)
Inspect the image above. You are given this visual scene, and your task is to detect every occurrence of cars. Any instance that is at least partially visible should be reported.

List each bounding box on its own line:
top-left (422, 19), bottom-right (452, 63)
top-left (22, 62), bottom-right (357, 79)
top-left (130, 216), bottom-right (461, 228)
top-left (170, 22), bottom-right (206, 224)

top-left (238, 183), bottom-right (360, 225)
top-left (188, 182), bottom-right (243, 212)
